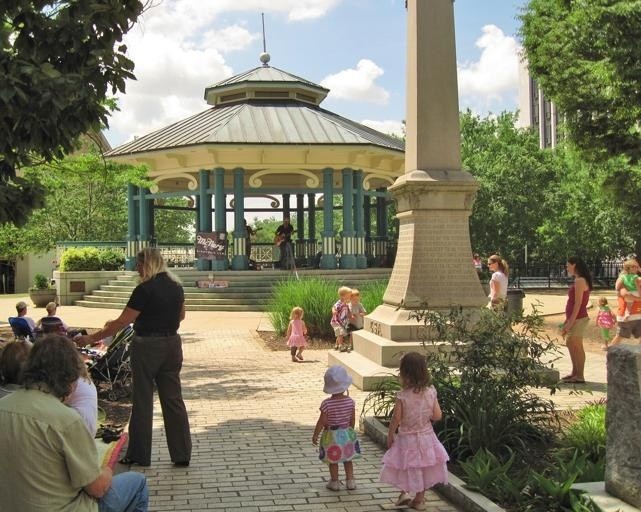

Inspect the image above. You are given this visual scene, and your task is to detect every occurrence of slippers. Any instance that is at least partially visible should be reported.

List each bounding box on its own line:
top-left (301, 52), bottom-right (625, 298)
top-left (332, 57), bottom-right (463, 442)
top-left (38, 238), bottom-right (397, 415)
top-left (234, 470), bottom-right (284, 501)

top-left (562, 376), bottom-right (585, 383)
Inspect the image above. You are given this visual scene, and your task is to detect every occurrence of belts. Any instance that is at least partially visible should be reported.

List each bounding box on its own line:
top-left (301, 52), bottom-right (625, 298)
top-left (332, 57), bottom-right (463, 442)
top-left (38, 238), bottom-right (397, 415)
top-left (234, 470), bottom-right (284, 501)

top-left (134, 330), bottom-right (177, 336)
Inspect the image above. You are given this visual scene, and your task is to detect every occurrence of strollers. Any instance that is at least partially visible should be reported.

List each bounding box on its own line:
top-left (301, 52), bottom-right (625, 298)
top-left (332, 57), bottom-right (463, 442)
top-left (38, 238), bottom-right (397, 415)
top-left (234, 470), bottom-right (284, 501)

top-left (81, 325), bottom-right (135, 401)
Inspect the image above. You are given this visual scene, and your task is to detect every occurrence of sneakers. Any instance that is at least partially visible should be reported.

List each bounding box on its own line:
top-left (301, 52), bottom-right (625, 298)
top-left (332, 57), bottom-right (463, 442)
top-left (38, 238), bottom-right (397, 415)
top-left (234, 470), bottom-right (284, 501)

top-left (325, 479), bottom-right (339, 490)
top-left (100, 422), bottom-right (124, 443)
top-left (118, 458), bottom-right (149, 465)
top-left (334, 342), bottom-right (353, 351)
top-left (395, 491), bottom-right (412, 506)
top-left (346, 480), bottom-right (355, 490)
top-left (292, 356), bottom-right (303, 361)
top-left (409, 499), bottom-right (426, 510)
top-left (176, 461), bottom-right (190, 466)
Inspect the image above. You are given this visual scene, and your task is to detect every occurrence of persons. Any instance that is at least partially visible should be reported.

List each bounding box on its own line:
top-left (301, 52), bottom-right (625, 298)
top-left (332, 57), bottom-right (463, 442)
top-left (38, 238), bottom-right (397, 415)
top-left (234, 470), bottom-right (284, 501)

top-left (473, 253), bottom-right (483, 269)
top-left (61, 354), bottom-right (99, 436)
top-left (379, 351), bottom-right (450, 511)
top-left (74, 248), bottom-right (192, 466)
top-left (487, 254), bottom-right (509, 309)
top-left (612, 258), bottom-right (641, 346)
top-left (313, 365), bottom-right (363, 489)
top-left (349, 289), bottom-right (367, 329)
top-left (559, 256), bottom-right (593, 383)
top-left (0, 339), bottom-right (34, 398)
top-left (276, 216), bottom-right (295, 261)
top-left (38, 301), bottom-right (72, 335)
top-left (18, 303), bottom-right (43, 335)
top-left (329, 286), bottom-right (356, 352)
top-left (244, 219), bottom-right (257, 258)
top-left (595, 296), bottom-right (618, 349)
top-left (0, 333), bottom-right (149, 512)
top-left (288, 307), bottom-right (308, 361)
top-left (620, 259), bottom-right (641, 321)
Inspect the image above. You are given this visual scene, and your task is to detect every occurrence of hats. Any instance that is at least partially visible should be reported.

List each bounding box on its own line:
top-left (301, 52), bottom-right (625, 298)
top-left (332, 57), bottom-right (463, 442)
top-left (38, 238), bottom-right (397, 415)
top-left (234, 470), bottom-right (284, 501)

top-left (46, 302), bottom-right (59, 311)
top-left (323, 366), bottom-right (352, 394)
top-left (16, 302), bottom-right (29, 310)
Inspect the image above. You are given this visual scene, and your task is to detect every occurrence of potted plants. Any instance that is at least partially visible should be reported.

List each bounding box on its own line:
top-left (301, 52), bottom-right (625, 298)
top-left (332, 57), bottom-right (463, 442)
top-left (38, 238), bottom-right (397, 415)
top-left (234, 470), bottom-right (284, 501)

top-left (27, 274), bottom-right (56, 308)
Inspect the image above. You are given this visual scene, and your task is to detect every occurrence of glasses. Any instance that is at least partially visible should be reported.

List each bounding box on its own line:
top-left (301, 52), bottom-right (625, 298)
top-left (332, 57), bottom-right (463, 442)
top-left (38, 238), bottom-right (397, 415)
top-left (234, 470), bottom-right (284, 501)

top-left (487, 262), bottom-right (496, 266)
